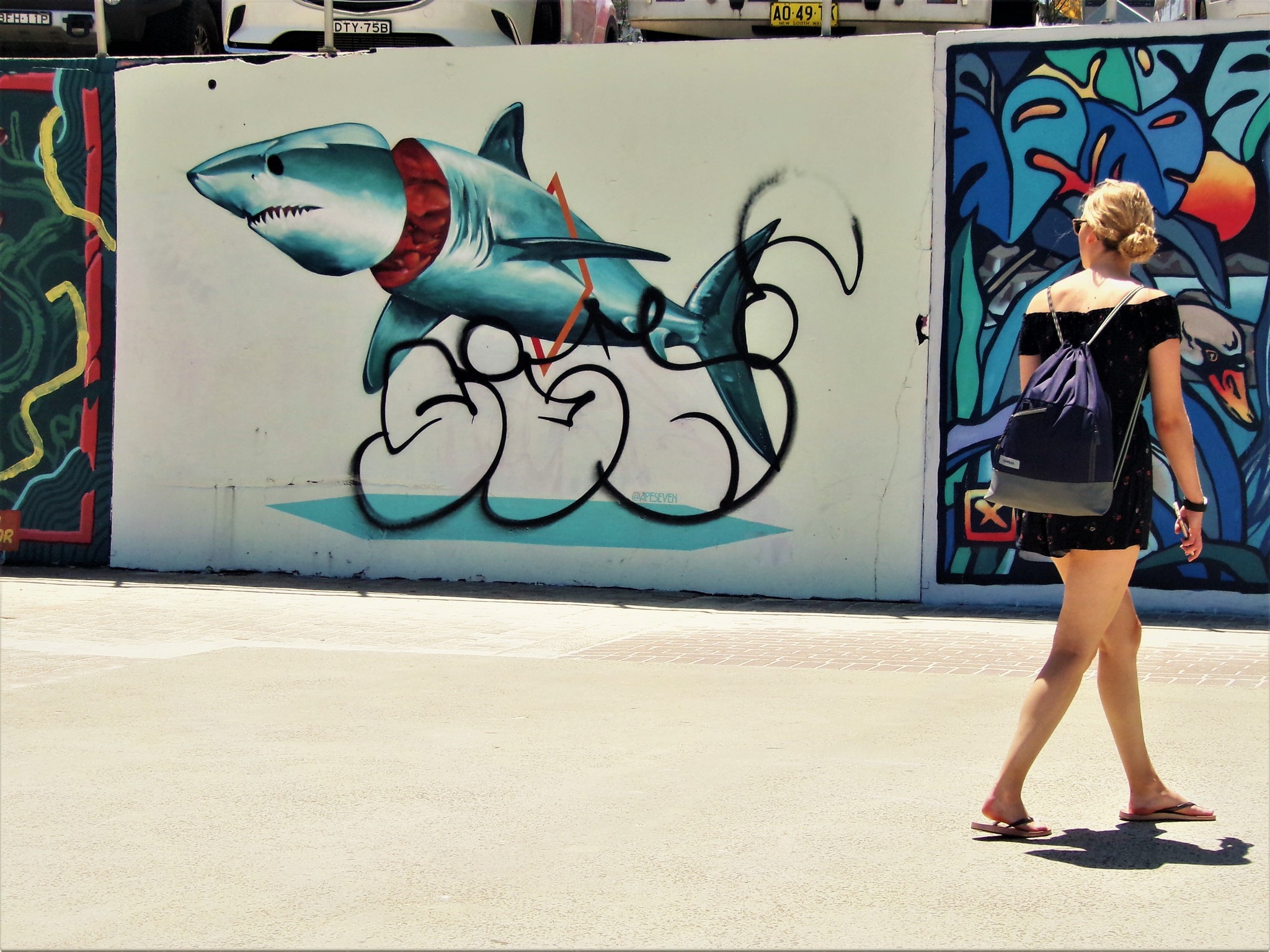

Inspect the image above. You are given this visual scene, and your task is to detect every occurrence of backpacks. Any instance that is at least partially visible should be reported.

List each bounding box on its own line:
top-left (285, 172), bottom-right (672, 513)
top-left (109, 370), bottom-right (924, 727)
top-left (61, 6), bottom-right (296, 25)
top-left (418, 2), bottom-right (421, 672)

top-left (983, 283), bottom-right (1149, 516)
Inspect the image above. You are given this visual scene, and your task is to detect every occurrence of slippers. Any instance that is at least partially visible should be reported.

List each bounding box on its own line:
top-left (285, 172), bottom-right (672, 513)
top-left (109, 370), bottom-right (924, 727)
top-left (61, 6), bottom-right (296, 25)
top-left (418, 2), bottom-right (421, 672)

top-left (1119, 801), bottom-right (1216, 821)
top-left (971, 817), bottom-right (1052, 837)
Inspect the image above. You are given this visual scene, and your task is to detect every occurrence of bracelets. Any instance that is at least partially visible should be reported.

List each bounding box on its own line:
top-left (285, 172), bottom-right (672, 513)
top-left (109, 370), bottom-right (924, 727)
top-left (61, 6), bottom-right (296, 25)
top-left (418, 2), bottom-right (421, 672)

top-left (1182, 492), bottom-right (1209, 513)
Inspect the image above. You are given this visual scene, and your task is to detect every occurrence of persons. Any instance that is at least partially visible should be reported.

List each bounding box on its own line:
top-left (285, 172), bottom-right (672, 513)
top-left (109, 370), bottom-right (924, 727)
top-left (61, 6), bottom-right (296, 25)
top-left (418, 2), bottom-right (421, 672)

top-left (972, 178), bottom-right (1219, 839)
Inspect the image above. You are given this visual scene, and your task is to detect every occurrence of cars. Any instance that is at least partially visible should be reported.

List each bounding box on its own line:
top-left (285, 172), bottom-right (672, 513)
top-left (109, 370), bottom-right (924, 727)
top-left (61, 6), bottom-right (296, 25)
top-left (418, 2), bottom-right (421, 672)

top-left (216, 1), bottom-right (623, 56)
top-left (620, 0), bottom-right (1044, 42)
top-left (0, 0), bottom-right (228, 64)
top-left (1139, 0), bottom-right (1270, 22)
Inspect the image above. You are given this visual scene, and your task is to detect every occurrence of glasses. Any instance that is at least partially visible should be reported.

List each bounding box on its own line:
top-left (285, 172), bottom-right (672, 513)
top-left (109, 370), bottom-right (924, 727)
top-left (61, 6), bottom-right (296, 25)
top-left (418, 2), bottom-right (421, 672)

top-left (1073, 218), bottom-right (1087, 236)
